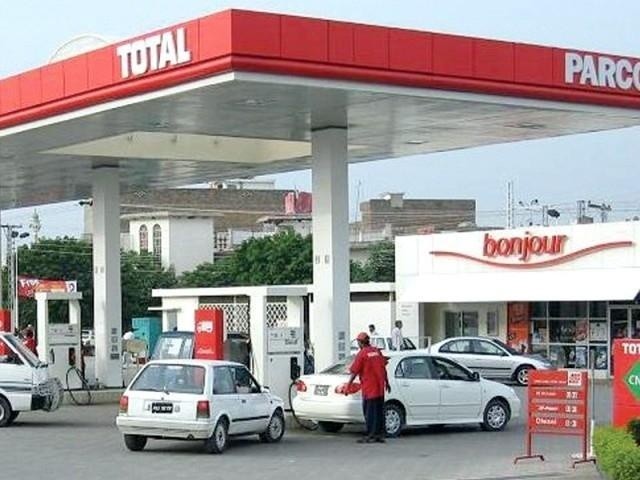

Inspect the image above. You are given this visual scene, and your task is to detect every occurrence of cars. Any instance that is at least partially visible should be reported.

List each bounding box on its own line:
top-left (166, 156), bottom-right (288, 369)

top-left (117, 359), bottom-right (286, 454)
top-left (419, 334), bottom-right (551, 387)
top-left (291, 348), bottom-right (523, 434)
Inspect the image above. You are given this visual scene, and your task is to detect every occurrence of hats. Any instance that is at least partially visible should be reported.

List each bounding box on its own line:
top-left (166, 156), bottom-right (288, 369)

top-left (357, 332), bottom-right (370, 340)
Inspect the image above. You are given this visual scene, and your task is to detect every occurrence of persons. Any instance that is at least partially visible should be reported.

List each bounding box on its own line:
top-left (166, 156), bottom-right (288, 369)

top-left (122, 326), bottom-right (135, 343)
top-left (342, 331), bottom-right (392, 445)
top-left (363, 324), bottom-right (385, 347)
top-left (389, 320), bottom-right (405, 352)
top-left (21, 329), bottom-right (38, 356)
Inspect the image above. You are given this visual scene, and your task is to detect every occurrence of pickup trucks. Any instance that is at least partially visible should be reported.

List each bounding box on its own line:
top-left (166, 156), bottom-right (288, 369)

top-left (1, 332), bottom-right (58, 426)
